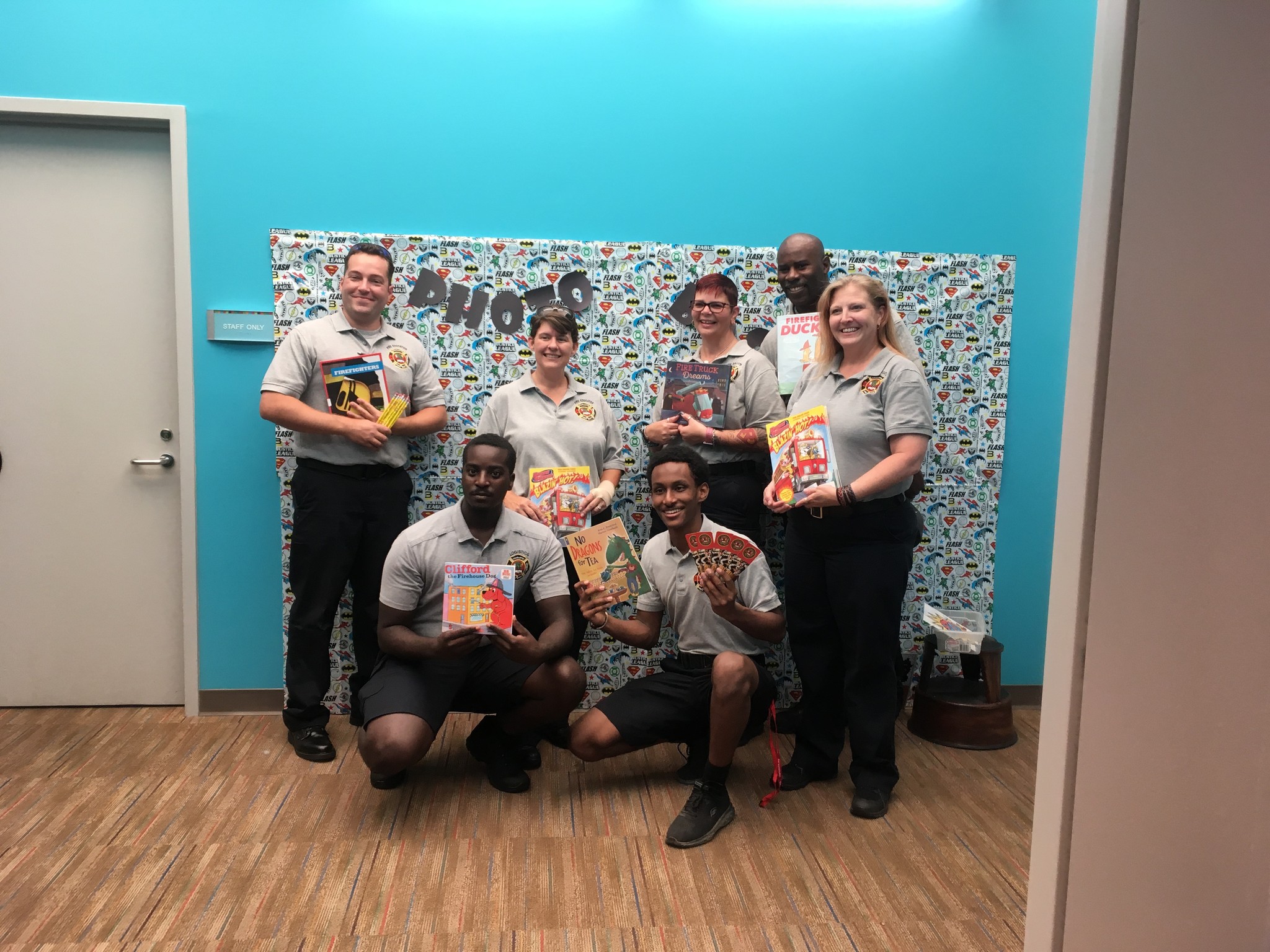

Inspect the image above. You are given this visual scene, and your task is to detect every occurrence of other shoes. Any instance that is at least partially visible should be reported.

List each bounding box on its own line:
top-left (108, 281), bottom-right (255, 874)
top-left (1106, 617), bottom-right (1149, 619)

top-left (770, 760), bottom-right (837, 791)
top-left (466, 735), bottom-right (532, 794)
top-left (850, 785), bottom-right (889, 819)
top-left (513, 737), bottom-right (542, 769)
top-left (546, 712), bottom-right (570, 747)
top-left (370, 770), bottom-right (404, 790)
top-left (771, 709), bottom-right (797, 734)
top-left (736, 721), bottom-right (765, 747)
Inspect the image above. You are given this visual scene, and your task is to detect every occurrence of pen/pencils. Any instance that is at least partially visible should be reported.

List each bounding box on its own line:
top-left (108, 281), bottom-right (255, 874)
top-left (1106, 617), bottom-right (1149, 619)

top-left (376, 392), bottom-right (411, 428)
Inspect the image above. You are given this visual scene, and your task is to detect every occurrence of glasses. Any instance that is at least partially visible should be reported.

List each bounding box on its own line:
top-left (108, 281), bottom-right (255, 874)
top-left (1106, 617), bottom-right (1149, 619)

top-left (535, 306), bottom-right (577, 320)
top-left (347, 243), bottom-right (392, 262)
top-left (690, 300), bottom-right (734, 313)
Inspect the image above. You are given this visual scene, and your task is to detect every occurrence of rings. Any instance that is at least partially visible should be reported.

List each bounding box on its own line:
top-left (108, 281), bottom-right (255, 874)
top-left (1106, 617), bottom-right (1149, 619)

top-left (597, 505), bottom-right (601, 510)
top-left (671, 435), bottom-right (676, 440)
top-left (804, 497), bottom-right (809, 505)
top-left (771, 509), bottom-right (774, 513)
top-left (687, 416), bottom-right (692, 421)
top-left (667, 417), bottom-right (670, 422)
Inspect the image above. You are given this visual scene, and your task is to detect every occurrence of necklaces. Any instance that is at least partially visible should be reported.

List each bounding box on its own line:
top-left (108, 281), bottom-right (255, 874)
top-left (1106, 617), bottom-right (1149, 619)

top-left (699, 337), bottom-right (739, 360)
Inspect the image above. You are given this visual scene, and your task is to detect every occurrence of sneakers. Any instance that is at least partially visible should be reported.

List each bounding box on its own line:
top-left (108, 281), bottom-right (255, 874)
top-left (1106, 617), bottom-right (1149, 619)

top-left (672, 741), bottom-right (709, 785)
top-left (664, 779), bottom-right (736, 849)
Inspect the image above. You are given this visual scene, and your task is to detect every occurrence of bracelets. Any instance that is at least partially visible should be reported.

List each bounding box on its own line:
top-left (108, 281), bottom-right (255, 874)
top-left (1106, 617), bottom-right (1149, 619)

top-left (590, 611), bottom-right (608, 630)
top-left (836, 484), bottom-right (857, 507)
top-left (712, 428), bottom-right (715, 445)
top-left (642, 424), bottom-right (659, 447)
top-left (703, 427), bottom-right (713, 445)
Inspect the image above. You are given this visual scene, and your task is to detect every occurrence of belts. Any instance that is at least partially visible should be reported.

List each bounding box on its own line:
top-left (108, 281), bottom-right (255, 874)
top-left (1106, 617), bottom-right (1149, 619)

top-left (801, 490), bottom-right (912, 519)
top-left (678, 654), bottom-right (765, 670)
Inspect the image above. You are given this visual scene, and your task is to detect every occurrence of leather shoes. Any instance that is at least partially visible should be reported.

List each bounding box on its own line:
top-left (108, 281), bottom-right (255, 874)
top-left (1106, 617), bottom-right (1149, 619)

top-left (288, 726), bottom-right (334, 762)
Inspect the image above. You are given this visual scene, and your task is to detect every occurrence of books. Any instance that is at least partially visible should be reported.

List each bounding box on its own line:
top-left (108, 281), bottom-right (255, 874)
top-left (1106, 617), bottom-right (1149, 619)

top-left (775, 312), bottom-right (837, 396)
top-left (442, 563), bottom-right (515, 638)
top-left (767, 405), bottom-right (844, 507)
top-left (659, 361), bottom-right (733, 431)
top-left (526, 466), bottom-right (592, 532)
top-left (563, 518), bottom-right (653, 611)
top-left (319, 354), bottom-right (391, 420)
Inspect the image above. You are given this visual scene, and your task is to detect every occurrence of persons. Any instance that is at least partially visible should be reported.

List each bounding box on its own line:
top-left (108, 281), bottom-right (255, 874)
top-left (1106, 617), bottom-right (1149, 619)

top-left (258, 242), bottom-right (447, 763)
top-left (569, 446), bottom-right (787, 848)
top-left (474, 306), bottom-right (627, 768)
top-left (757, 232), bottom-right (929, 736)
top-left (359, 434), bottom-right (587, 793)
top-left (764, 273), bottom-right (935, 821)
top-left (640, 275), bottom-right (787, 749)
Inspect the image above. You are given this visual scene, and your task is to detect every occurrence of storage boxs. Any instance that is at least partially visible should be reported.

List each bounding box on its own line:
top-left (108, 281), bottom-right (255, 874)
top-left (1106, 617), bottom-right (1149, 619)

top-left (930, 609), bottom-right (987, 655)
